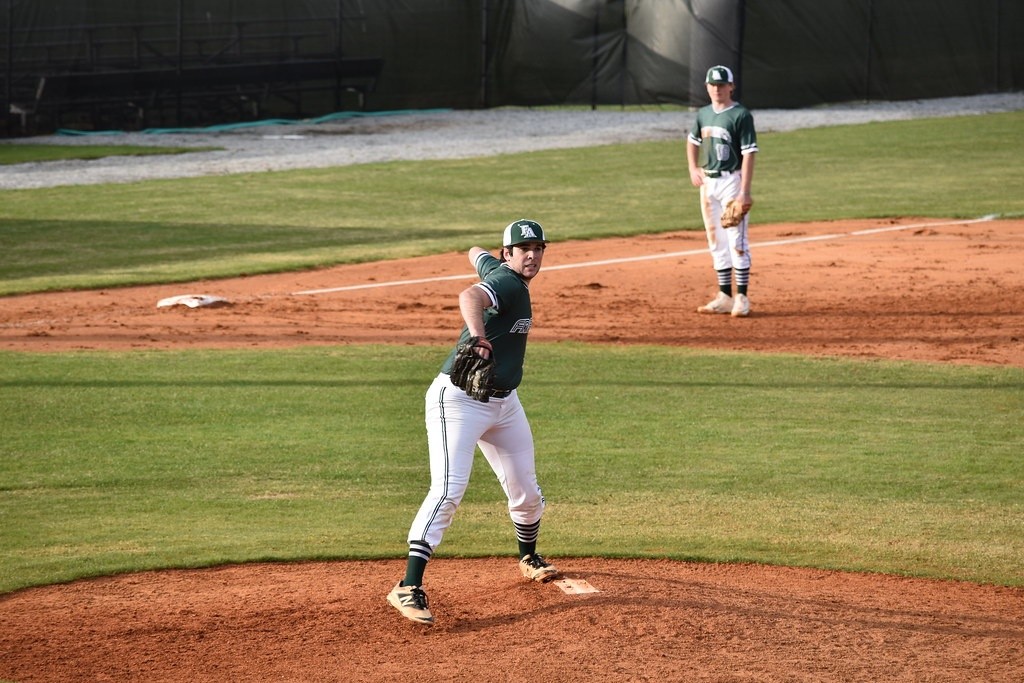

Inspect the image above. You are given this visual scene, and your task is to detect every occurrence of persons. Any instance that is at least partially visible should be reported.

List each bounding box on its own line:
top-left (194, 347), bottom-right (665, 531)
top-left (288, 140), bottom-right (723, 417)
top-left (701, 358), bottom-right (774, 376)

top-left (687, 65), bottom-right (759, 317)
top-left (386, 220), bottom-right (557, 626)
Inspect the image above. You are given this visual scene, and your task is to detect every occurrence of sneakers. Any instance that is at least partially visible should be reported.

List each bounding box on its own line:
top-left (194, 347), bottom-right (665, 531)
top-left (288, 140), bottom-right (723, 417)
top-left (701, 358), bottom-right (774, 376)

top-left (388, 581), bottom-right (433, 626)
top-left (698, 290), bottom-right (733, 313)
top-left (731, 293), bottom-right (750, 317)
top-left (518, 553), bottom-right (558, 583)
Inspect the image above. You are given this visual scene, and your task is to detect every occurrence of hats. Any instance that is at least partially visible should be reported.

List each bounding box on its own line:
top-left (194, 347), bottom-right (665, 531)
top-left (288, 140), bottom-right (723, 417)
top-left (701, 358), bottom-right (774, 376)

top-left (705, 64), bottom-right (733, 84)
top-left (502, 221), bottom-right (550, 248)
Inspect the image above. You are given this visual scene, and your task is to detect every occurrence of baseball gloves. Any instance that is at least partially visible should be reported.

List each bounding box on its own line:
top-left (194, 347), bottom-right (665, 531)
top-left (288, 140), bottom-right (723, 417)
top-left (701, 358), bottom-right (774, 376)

top-left (721, 199), bottom-right (754, 230)
top-left (449, 335), bottom-right (497, 403)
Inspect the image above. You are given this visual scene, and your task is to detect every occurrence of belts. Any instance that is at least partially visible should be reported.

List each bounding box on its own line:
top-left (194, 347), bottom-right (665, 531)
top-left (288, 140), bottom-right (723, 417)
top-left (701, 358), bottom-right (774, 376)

top-left (489, 389), bottom-right (511, 398)
top-left (705, 171), bottom-right (733, 178)
